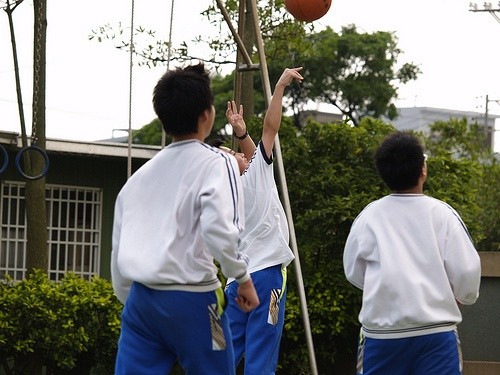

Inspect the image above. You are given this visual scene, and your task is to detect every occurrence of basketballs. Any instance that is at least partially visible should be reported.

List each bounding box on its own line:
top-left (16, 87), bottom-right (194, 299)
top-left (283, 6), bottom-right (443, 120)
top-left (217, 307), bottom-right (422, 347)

top-left (284, 0), bottom-right (333, 24)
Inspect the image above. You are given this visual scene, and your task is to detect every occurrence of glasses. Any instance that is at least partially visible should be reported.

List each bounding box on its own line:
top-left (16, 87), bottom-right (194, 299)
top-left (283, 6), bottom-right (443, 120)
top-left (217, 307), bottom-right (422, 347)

top-left (423, 153), bottom-right (428, 163)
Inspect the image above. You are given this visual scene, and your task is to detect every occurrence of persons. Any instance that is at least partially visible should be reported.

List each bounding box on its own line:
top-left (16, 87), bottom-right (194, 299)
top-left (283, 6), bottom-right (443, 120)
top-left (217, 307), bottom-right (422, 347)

top-left (111, 60), bottom-right (303, 375)
top-left (343, 129), bottom-right (481, 375)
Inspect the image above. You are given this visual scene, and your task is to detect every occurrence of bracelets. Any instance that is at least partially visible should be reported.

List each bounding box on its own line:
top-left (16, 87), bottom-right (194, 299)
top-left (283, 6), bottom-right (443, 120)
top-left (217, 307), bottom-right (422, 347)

top-left (233, 130), bottom-right (249, 141)
top-left (235, 269), bottom-right (250, 284)
